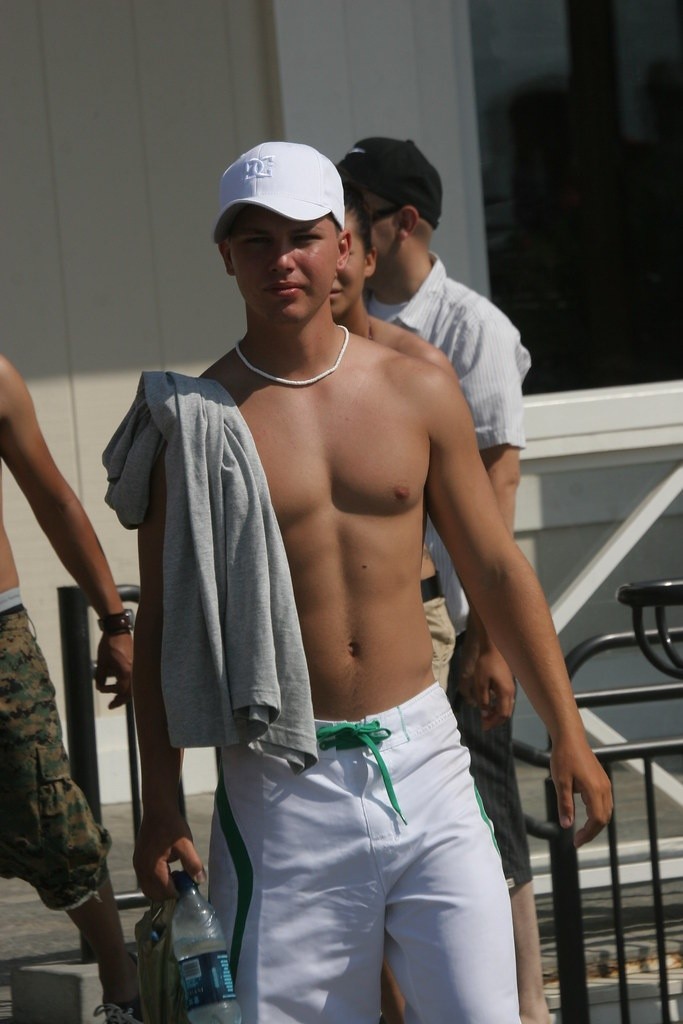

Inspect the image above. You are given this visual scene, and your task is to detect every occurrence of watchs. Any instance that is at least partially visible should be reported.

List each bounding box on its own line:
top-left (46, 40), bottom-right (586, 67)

top-left (96, 609), bottom-right (135, 634)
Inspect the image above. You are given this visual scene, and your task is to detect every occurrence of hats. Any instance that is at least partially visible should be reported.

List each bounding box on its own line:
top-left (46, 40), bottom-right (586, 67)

top-left (336, 136), bottom-right (442, 229)
top-left (211, 141), bottom-right (345, 243)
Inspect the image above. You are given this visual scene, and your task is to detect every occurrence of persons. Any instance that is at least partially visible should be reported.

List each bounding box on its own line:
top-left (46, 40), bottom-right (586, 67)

top-left (337, 138), bottom-right (553, 1024)
top-left (127, 141), bottom-right (615, 1024)
top-left (0, 359), bottom-right (143, 1024)
top-left (328, 184), bottom-right (455, 1024)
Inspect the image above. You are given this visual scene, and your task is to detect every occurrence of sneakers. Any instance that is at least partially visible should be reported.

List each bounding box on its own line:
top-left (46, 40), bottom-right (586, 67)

top-left (92, 949), bottom-right (144, 1024)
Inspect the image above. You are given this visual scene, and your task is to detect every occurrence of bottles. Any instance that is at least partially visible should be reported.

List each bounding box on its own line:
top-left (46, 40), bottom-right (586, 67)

top-left (169, 878), bottom-right (242, 1024)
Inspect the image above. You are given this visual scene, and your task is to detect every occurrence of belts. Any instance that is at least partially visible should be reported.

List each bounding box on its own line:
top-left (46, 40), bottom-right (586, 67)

top-left (420, 568), bottom-right (444, 604)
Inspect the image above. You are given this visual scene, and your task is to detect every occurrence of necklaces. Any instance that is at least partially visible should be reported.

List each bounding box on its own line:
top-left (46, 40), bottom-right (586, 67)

top-left (235, 325), bottom-right (349, 387)
